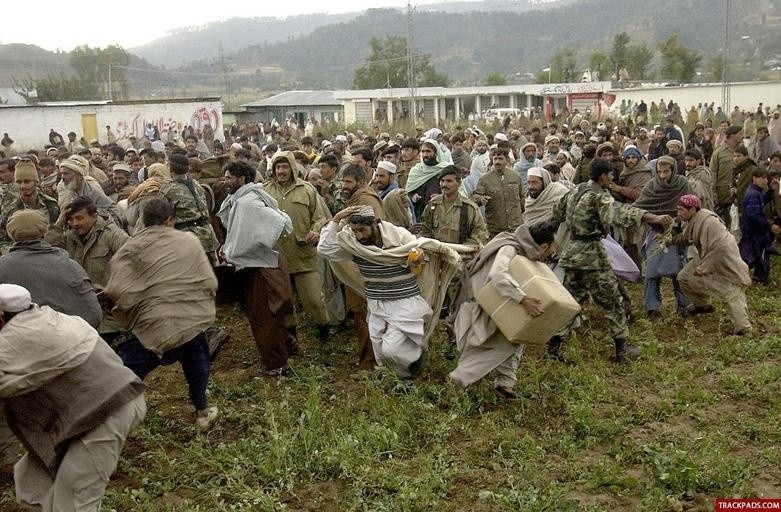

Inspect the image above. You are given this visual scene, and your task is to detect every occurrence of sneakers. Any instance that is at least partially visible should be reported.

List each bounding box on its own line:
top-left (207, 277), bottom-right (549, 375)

top-left (209, 331), bottom-right (231, 361)
top-left (194, 406), bottom-right (220, 432)
top-left (687, 304), bottom-right (715, 313)
top-left (495, 384), bottom-right (519, 401)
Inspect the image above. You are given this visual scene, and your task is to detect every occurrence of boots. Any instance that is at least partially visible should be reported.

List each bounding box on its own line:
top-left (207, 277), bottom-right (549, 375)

top-left (614, 337), bottom-right (641, 361)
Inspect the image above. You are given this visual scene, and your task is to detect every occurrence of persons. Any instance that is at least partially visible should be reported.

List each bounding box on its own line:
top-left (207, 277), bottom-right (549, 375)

top-left (551, 158), bottom-right (673, 364)
top-left (131, 124), bottom-right (228, 200)
top-left (0, 283), bottom-right (147, 510)
top-left (659, 194), bottom-right (753, 340)
top-left (406, 139), bottom-right (458, 226)
top-left (448, 220), bottom-right (559, 403)
top-left (374, 160), bottom-right (417, 241)
top-left (524, 167), bottom-right (571, 270)
top-left (264, 150), bottom-right (333, 354)
top-left (628, 154), bottom-right (694, 320)
top-left (470, 147), bottom-right (525, 255)
top-left (333, 109), bottom-right (393, 169)
top-left (299, 114), bottom-right (333, 190)
top-left (47, 195), bottom-right (132, 343)
top-left (709, 125), bottom-right (750, 231)
top-left (58, 125), bottom-right (131, 217)
top-left (318, 157), bottom-right (347, 218)
top-left (393, 106), bottom-right (424, 188)
top-left (1, 130), bottom-right (29, 213)
top-left (727, 103), bottom-right (781, 166)
top-left (101, 198), bottom-right (219, 430)
top-left (0, 158), bottom-right (57, 252)
top-left (316, 204), bottom-right (460, 397)
top-left (729, 148), bottom-right (772, 231)
top-left (500, 100), bottom-right (684, 185)
top-left (330, 164), bottom-right (385, 347)
top-left (741, 167), bottom-right (781, 294)
top-left (160, 153), bottom-right (228, 359)
top-left (424, 128), bottom-right (458, 166)
top-left (417, 166), bottom-right (489, 362)
top-left (683, 150), bottom-right (713, 221)
top-left (687, 103), bottom-right (727, 166)
top-left (771, 153), bottom-right (781, 229)
top-left (222, 160), bottom-right (300, 380)
top-left (609, 145), bottom-right (653, 291)
top-left (458, 108), bottom-right (501, 194)
top-left (29, 129), bottom-right (58, 197)
top-left (228, 113), bottom-right (300, 181)
top-left (1, 209), bottom-right (103, 331)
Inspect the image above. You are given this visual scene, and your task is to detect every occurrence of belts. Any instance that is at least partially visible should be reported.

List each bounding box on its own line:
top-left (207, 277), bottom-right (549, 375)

top-left (175, 217), bottom-right (209, 228)
top-left (569, 234), bottom-right (602, 240)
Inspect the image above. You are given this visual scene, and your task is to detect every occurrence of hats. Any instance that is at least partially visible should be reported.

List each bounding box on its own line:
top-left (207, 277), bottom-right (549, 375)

top-left (666, 139), bottom-right (683, 148)
top-left (336, 134), bottom-right (348, 141)
top-left (576, 131), bottom-right (584, 135)
top-left (625, 144), bottom-right (636, 150)
top-left (232, 142), bottom-right (242, 150)
top-left (473, 132), bottom-right (479, 137)
top-left (6, 208), bottom-right (50, 241)
top-left (349, 205), bottom-right (375, 217)
top-left (14, 158), bottom-right (40, 182)
top-left (0, 283), bottom-right (31, 313)
top-left (90, 148), bottom-right (101, 153)
top-left (113, 164), bottom-right (131, 171)
top-left (61, 155), bottom-right (87, 176)
top-left (321, 139), bottom-right (332, 149)
top-left (527, 167), bottom-right (543, 178)
top-left (127, 147), bottom-right (137, 153)
top-left (437, 165), bottom-right (461, 184)
top-left (382, 133), bottom-right (389, 138)
top-left (373, 140), bottom-right (387, 150)
top-left (562, 124), bottom-right (568, 128)
top-left (589, 136), bottom-right (599, 142)
top-left (545, 135), bottom-right (560, 144)
top-left (596, 143), bottom-right (613, 156)
top-left (424, 138), bottom-right (438, 151)
top-left (66, 195), bottom-right (94, 216)
top-left (724, 125), bottom-right (741, 135)
top-left (169, 153), bottom-right (190, 166)
top-left (658, 156), bottom-right (675, 165)
top-left (495, 132), bottom-right (508, 141)
top-left (380, 144), bottom-right (399, 157)
top-left (318, 155), bottom-right (336, 164)
top-left (140, 147), bottom-right (153, 156)
top-left (47, 147), bottom-right (58, 155)
top-left (378, 161), bottom-right (397, 174)
top-left (560, 150), bottom-right (570, 158)
top-left (677, 194), bottom-right (702, 209)
